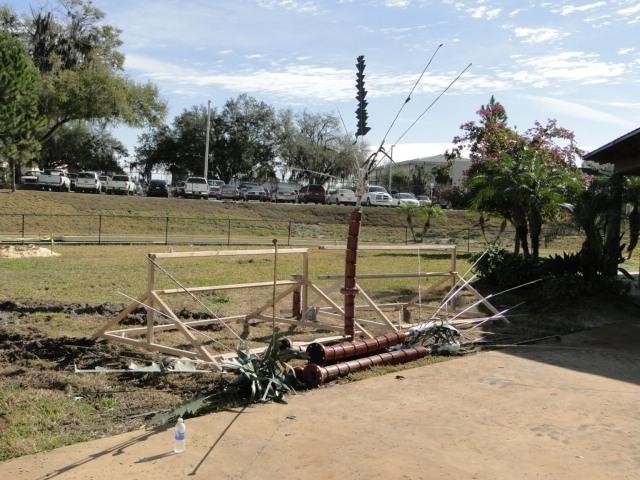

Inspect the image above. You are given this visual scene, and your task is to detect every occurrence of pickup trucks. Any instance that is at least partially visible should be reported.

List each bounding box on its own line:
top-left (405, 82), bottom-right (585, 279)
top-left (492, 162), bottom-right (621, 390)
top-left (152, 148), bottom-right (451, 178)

top-left (36, 169), bottom-right (71, 192)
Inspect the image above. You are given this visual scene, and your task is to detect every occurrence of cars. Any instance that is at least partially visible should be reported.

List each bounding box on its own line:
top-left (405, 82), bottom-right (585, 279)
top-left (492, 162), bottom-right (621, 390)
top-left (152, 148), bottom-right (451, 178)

top-left (65, 171), bottom-right (267, 203)
top-left (360, 185), bottom-right (393, 207)
top-left (325, 189), bottom-right (337, 205)
top-left (270, 185), bottom-right (298, 205)
top-left (416, 195), bottom-right (432, 207)
top-left (328, 188), bottom-right (358, 207)
top-left (393, 192), bottom-right (420, 208)
top-left (20, 170), bottom-right (41, 185)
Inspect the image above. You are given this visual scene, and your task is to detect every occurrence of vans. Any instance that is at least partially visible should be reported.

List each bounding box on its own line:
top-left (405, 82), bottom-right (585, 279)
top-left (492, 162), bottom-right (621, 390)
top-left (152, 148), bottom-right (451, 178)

top-left (297, 184), bottom-right (325, 205)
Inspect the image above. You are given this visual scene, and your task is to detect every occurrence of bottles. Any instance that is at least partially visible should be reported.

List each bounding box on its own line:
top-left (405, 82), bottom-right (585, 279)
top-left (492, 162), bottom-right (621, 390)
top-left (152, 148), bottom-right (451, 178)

top-left (173, 417), bottom-right (186, 454)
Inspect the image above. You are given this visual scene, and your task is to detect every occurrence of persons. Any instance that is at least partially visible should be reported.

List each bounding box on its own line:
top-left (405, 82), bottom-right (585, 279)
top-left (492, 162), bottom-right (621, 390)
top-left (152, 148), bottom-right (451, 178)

top-left (127, 180), bottom-right (148, 198)
